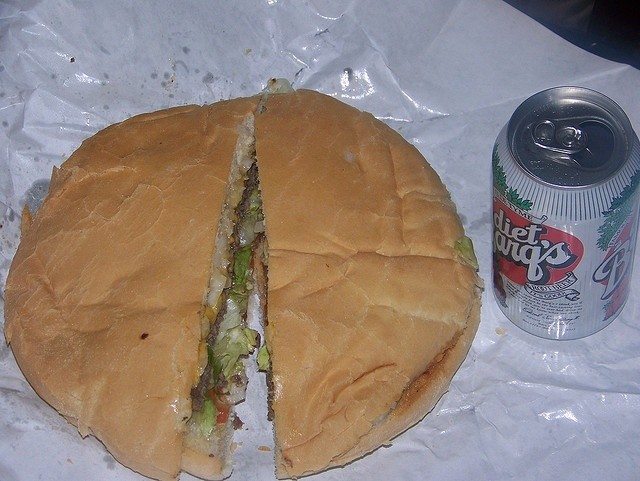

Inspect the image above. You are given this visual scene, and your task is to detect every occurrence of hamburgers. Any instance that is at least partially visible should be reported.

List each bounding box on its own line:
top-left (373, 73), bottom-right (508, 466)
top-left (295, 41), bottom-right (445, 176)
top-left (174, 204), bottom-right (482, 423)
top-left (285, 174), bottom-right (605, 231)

top-left (4, 78), bottom-right (484, 477)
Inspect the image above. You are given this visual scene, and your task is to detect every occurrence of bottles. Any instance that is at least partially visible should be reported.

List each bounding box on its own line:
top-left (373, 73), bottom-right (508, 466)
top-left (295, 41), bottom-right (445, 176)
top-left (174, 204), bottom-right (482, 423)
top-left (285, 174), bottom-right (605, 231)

top-left (492, 86), bottom-right (639, 342)
